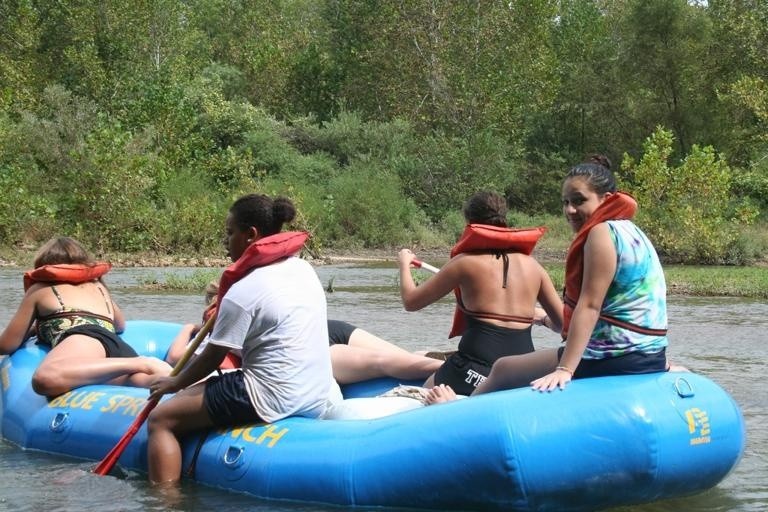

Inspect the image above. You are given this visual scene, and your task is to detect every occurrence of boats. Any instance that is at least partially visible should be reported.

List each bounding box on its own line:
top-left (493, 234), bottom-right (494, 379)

top-left (1, 309), bottom-right (749, 509)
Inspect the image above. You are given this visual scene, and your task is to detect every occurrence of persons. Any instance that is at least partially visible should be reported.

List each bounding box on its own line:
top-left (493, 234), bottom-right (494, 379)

top-left (142, 191), bottom-right (347, 505)
top-left (0, 230), bottom-right (175, 400)
top-left (396, 189), bottom-right (566, 407)
top-left (162, 269), bottom-right (451, 390)
top-left (424, 151), bottom-right (668, 406)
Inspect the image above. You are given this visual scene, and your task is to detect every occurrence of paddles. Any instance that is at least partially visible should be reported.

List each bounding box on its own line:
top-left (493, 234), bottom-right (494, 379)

top-left (92, 310), bottom-right (217, 478)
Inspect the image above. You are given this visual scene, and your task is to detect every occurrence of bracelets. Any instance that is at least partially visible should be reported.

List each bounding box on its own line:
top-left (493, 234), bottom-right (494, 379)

top-left (554, 366), bottom-right (575, 378)
